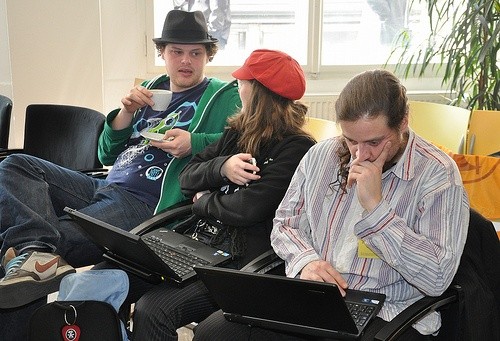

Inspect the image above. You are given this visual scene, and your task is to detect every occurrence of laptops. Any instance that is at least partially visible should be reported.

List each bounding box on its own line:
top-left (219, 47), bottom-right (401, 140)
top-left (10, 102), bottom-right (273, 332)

top-left (63, 207), bottom-right (234, 287)
top-left (193, 265), bottom-right (386, 341)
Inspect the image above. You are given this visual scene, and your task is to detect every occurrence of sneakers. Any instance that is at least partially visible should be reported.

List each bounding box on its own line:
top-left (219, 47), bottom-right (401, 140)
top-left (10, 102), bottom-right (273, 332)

top-left (0, 250), bottom-right (76, 309)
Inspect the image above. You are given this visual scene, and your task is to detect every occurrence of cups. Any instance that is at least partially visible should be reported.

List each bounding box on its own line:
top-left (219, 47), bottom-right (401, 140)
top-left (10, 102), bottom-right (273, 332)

top-left (148, 90), bottom-right (172, 111)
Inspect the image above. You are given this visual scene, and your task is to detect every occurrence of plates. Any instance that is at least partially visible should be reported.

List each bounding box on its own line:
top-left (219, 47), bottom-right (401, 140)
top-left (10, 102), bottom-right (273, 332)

top-left (140, 132), bottom-right (174, 143)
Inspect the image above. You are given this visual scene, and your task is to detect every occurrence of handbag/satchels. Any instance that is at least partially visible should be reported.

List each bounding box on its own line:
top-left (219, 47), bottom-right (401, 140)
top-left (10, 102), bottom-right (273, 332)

top-left (0, 271), bottom-right (133, 341)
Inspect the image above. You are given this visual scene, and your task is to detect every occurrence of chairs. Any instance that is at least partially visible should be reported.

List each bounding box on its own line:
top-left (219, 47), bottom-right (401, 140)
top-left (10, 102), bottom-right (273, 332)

top-left (240, 205), bottom-right (500, 341)
top-left (0, 96), bottom-right (109, 180)
top-left (299, 101), bottom-right (500, 218)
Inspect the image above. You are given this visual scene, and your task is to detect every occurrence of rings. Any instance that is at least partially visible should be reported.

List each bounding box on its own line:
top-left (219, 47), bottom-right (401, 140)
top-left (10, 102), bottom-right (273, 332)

top-left (240, 162), bottom-right (246, 168)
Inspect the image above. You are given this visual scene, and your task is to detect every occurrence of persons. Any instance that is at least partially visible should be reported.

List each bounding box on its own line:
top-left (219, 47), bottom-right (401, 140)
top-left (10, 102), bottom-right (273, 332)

top-left (193, 69), bottom-right (470, 341)
top-left (89, 48), bottom-right (317, 341)
top-left (0, 10), bottom-right (244, 310)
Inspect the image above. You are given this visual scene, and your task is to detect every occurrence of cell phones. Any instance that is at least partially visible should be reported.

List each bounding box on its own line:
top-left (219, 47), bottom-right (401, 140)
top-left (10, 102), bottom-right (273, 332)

top-left (244, 158), bottom-right (257, 174)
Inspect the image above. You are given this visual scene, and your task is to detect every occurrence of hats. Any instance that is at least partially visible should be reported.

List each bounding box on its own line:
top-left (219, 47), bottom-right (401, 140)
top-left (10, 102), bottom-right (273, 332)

top-left (231, 49), bottom-right (306, 101)
top-left (152, 9), bottom-right (219, 44)
